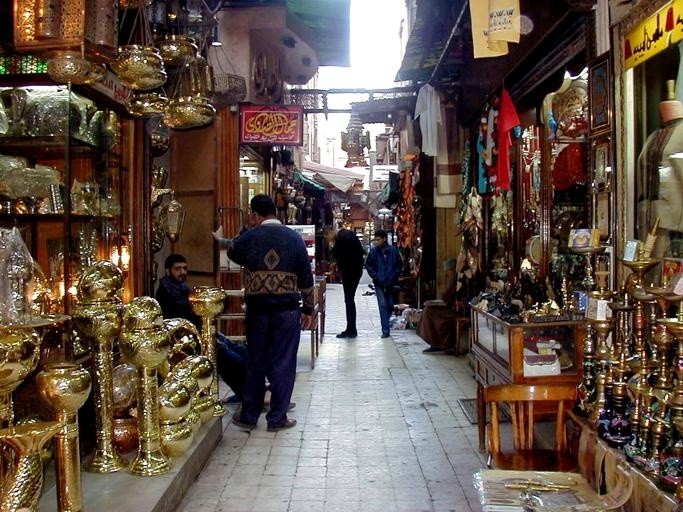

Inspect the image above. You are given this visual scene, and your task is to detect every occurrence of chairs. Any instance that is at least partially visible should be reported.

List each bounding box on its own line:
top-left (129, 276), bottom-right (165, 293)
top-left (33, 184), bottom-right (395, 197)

top-left (481, 382), bottom-right (580, 471)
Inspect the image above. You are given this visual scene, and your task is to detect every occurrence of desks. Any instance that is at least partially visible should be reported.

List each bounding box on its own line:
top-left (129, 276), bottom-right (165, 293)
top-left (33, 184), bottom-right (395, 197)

top-left (300, 275), bottom-right (329, 371)
top-left (471, 467), bottom-right (615, 512)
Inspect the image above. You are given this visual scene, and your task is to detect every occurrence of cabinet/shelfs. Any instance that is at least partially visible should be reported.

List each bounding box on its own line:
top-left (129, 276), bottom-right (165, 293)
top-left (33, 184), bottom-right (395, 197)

top-left (565, 407), bottom-right (683, 512)
top-left (0, 74), bottom-right (140, 463)
top-left (464, 300), bottom-right (586, 424)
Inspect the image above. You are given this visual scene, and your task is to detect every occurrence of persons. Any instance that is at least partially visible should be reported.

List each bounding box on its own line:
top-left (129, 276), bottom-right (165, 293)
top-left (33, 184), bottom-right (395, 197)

top-left (226, 193), bottom-right (314, 430)
top-left (212, 226), bottom-right (239, 250)
top-left (366, 231), bottom-right (403, 337)
top-left (637, 99), bottom-right (683, 266)
top-left (331, 223), bottom-right (364, 338)
top-left (156, 255), bottom-right (272, 405)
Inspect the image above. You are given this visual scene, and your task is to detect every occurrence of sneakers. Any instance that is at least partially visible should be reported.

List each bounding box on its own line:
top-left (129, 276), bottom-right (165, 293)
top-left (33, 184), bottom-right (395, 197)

top-left (232, 412), bottom-right (257, 429)
top-left (423, 347), bottom-right (445, 354)
top-left (336, 331), bottom-right (357, 338)
top-left (266, 418), bottom-right (297, 431)
